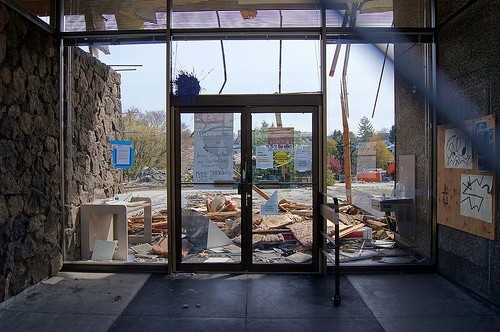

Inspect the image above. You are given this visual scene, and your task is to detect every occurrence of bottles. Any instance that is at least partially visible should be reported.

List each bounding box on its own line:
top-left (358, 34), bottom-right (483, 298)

top-left (115, 194), bottom-right (119, 201)
